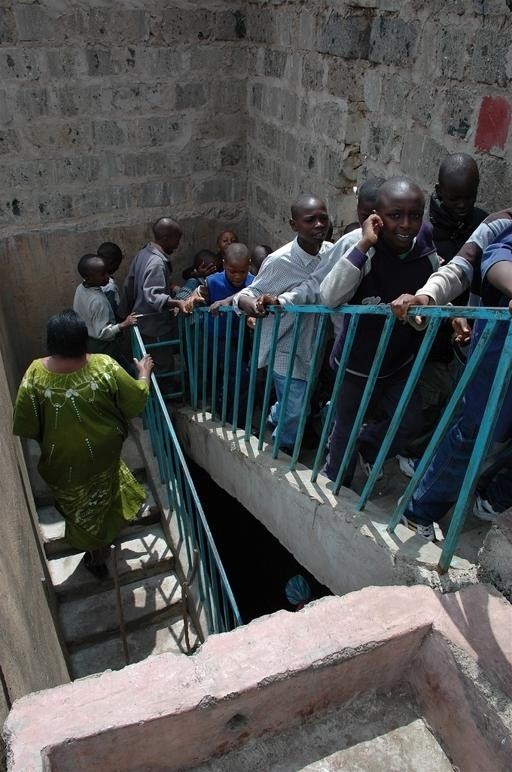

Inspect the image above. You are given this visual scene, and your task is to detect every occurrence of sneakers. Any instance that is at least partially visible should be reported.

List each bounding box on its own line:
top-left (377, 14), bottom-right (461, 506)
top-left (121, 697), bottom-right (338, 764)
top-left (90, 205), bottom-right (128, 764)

top-left (473, 492), bottom-right (500, 524)
top-left (322, 399), bottom-right (338, 450)
top-left (398, 495), bottom-right (434, 543)
top-left (238, 426), bottom-right (262, 439)
top-left (356, 423), bottom-right (385, 482)
top-left (279, 445), bottom-right (304, 463)
top-left (395, 451), bottom-right (424, 480)
top-left (267, 415), bottom-right (282, 430)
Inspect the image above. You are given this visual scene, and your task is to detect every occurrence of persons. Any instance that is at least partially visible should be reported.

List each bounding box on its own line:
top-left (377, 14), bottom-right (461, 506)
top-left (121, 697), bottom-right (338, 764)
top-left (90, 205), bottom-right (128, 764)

top-left (122, 216), bottom-right (192, 393)
top-left (173, 249), bottom-right (222, 316)
top-left (184, 241), bottom-right (256, 417)
top-left (12, 310), bottom-right (154, 580)
top-left (73, 253), bottom-right (138, 355)
top-left (256, 176), bottom-right (386, 369)
top-left (249, 244), bottom-right (273, 277)
top-left (320, 176), bottom-right (473, 488)
top-left (398, 228), bottom-right (511, 543)
top-left (182, 230), bottom-right (237, 280)
top-left (391, 208), bottom-right (511, 324)
top-left (424, 152), bottom-right (492, 364)
top-left (232, 195), bottom-right (334, 458)
top-left (70, 242), bottom-right (123, 321)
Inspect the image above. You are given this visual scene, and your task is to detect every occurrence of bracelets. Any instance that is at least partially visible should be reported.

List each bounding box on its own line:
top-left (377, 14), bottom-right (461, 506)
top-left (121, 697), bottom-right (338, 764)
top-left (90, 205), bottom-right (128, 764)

top-left (138, 376), bottom-right (151, 383)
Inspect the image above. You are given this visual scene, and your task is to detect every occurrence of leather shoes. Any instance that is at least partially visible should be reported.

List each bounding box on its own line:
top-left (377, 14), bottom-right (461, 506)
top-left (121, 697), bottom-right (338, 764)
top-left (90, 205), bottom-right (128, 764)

top-left (83, 553), bottom-right (108, 580)
